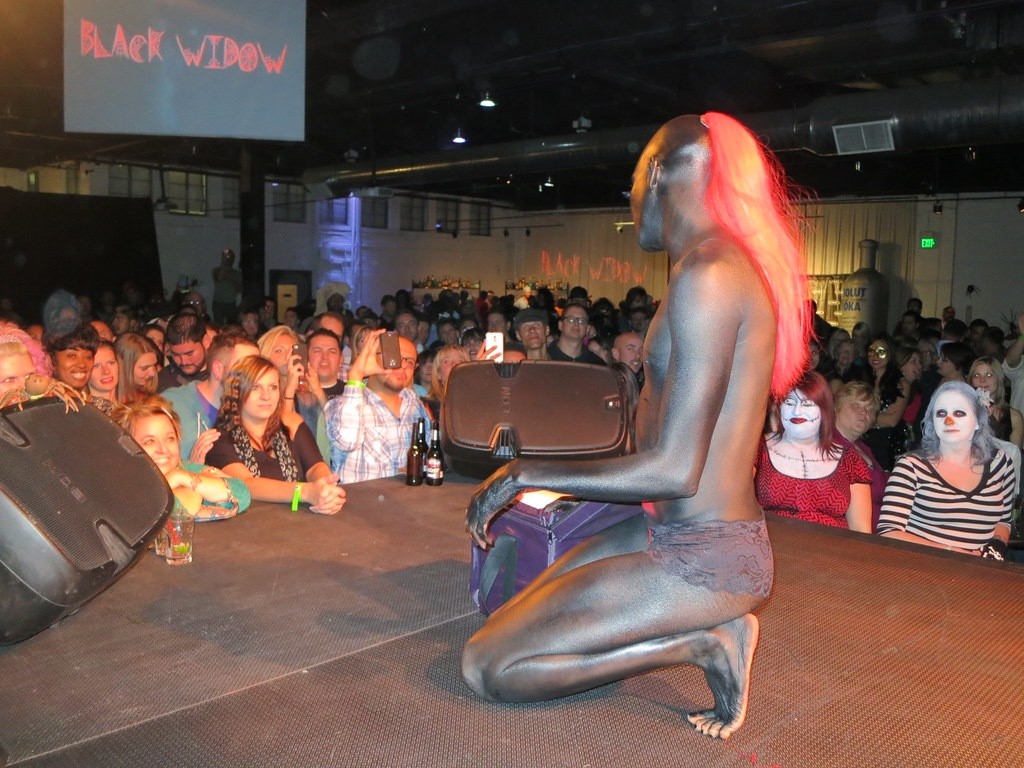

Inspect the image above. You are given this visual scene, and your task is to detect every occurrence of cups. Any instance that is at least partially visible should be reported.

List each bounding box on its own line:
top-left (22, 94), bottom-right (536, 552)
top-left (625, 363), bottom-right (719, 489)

top-left (153, 502), bottom-right (195, 566)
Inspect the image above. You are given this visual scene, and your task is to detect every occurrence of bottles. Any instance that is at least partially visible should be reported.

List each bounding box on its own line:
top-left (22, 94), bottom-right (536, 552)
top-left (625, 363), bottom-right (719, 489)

top-left (418, 272), bottom-right (479, 289)
top-left (406, 423), bottom-right (422, 485)
top-left (426, 422), bottom-right (443, 486)
top-left (417, 417), bottom-right (429, 478)
top-left (1010, 493), bottom-right (1024, 540)
top-left (505, 273), bottom-right (567, 291)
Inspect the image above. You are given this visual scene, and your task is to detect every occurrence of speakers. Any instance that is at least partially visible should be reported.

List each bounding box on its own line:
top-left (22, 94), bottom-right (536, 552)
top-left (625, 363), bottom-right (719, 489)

top-left (0, 395), bottom-right (174, 646)
top-left (438, 360), bottom-right (640, 479)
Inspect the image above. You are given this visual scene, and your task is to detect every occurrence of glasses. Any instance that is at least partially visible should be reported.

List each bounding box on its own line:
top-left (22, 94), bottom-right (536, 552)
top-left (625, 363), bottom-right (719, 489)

top-left (562, 316), bottom-right (589, 324)
top-left (401, 357), bottom-right (416, 369)
top-left (186, 300), bottom-right (204, 306)
top-left (241, 320), bottom-right (257, 324)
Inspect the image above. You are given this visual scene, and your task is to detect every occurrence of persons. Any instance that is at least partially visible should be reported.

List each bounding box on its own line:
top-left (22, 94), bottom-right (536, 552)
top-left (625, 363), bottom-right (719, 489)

top-left (0, 280), bottom-right (1024, 539)
top-left (212, 249), bottom-right (241, 324)
top-left (877, 380), bottom-right (1016, 561)
top-left (111, 392), bottom-right (249, 521)
top-left (324, 328), bottom-right (432, 485)
top-left (204, 356), bottom-right (347, 515)
top-left (751, 372), bottom-right (871, 533)
top-left (461, 113), bottom-right (814, 740)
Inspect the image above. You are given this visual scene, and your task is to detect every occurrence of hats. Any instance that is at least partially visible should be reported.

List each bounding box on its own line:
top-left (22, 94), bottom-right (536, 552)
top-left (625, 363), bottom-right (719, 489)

top-left (512, 309), bottom-right (549, 330)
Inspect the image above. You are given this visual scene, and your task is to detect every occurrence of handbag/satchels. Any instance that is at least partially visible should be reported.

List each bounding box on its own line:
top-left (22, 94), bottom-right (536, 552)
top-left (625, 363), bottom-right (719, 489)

top-left (470, 490), bottom-right (644, 617)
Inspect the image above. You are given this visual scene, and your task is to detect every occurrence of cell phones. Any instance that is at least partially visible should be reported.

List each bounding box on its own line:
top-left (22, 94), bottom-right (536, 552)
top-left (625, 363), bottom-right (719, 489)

top-left (292, 344), bottom-right (307, 375)
top-left (380, 332), bottom-right (401, 370)
top-left (486, 332), bottom-right (504, 363)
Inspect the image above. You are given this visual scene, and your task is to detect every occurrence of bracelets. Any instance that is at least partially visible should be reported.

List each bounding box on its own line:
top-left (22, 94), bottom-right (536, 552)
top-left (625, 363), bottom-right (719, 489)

top-left (190, 473), bottom-right (201, 489)
top-left (347, 380), bottom-right (364, 389)
top-left (284, 397), bottom-right (294, 400)
top-left (292, 482), bottom-right (303, 511)
